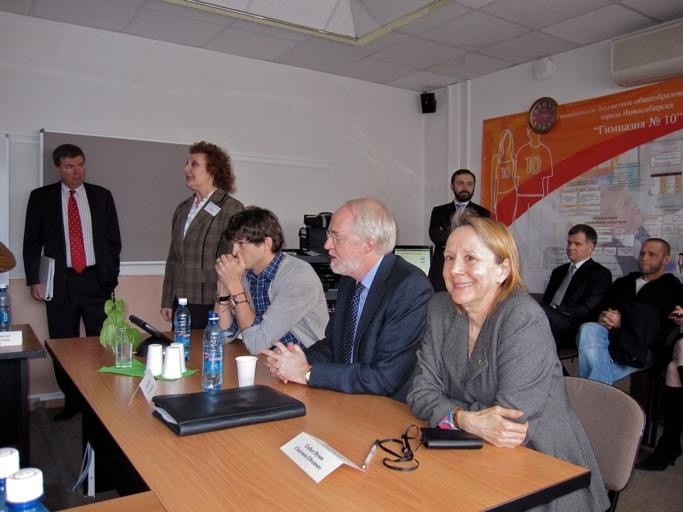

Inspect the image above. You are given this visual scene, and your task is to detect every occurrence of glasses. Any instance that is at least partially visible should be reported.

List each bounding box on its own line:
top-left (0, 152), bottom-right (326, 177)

top-left (325, 231), bottom-right (345, 241)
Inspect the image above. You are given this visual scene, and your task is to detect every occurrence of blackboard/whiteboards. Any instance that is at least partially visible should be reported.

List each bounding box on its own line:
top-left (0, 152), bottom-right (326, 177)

top-left (37, 128), bottom-right (216, 266)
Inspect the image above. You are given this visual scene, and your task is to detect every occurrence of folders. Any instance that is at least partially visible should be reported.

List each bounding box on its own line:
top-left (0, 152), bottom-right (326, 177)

top-left (38, 255), bottom-right (57, 301)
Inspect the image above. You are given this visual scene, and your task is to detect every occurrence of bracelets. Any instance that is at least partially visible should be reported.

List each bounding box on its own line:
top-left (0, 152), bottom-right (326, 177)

top-left (229, 292), bottom-right (249, 309)
top-left (452, 408), bottom-right (463, 429)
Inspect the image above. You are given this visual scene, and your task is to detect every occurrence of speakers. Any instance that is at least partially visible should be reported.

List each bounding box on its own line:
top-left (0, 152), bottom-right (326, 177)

top-left (421, 91), bottom-right (437, 114)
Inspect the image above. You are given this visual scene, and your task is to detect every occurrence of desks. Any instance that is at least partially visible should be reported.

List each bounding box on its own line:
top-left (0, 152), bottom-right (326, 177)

top-left (0, 324), bottom-right (46, 467)
top-left (44, 321), bottom-right (592, 512)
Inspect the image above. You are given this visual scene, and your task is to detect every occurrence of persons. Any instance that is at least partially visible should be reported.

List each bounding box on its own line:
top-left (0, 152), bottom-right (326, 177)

top-left (407, 214), bottom-right (612, 511)
top-left (575, 238), bottom-right (682, 471)
top-left (24, 145), bottom-right (122, 422)
top-left (0, 240), bottom-right (17, 276)
top-left (427, 171), bottom-right (490, 292)
top-left (259, 196), bottom-right (435, 405)
top-left (539, 223), bottom-right (612, 345)
top-left (160, 142), bottom-right (246, 331)
top-left (212, 207), bottom-right (331, 354)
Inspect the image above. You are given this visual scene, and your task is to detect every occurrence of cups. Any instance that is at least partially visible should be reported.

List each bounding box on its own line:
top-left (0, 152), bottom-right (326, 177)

top-left (235, 355), bottom-right (258, 388)
top-left (161, 346), bottom-right (183, 380)
top-left (144, 344), bottom-right (164, 377)
top-left (114, 328), bottom-right (132, 369)
top-left (170, 342), bottom-right (187, 374)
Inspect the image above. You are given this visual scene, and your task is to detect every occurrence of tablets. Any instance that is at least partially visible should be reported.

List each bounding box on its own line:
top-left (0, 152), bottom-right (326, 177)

top-left (128, 314), bottom-right (171, 341)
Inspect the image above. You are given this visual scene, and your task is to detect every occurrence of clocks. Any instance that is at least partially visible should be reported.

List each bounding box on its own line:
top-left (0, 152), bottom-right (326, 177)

top-left (528, 96), bottom-right (559, 134)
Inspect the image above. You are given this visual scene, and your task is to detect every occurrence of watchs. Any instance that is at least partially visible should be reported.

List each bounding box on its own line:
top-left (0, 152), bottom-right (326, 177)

top-left (214, 291), bottom-right (231, 306)
top-left (304, 369), bottom-right (311, 384)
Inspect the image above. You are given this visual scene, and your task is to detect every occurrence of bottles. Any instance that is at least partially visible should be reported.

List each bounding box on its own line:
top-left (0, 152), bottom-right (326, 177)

top-left (0, 283), bottom-right (12, 332)
top-left (0, 447), bottom-right (20, 512)
top-left (2, 467), bottom-right (50, 512)
top-left (201, 311), bottom-right (224, 392)
top-left (173, 297), bottom-right (192, 363)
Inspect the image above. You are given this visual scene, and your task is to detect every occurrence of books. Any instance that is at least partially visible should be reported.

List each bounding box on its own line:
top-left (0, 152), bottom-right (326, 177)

top-left (420, 427), bottom-right (483, 450)
top-left (152, 386), bottom-right (305, 434)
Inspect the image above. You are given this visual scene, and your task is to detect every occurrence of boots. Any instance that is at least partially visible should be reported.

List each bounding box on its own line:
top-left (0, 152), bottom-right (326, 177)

top-left (636, 385), bottom-right (683, 470)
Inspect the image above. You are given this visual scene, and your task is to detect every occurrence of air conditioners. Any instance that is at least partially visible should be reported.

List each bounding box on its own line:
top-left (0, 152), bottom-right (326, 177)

top-left (609, 17), bottom-right (683, 87)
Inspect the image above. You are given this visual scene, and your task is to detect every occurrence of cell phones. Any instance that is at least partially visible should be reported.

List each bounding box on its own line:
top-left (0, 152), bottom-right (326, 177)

top-left (674, 312), bottom-right (682, 318)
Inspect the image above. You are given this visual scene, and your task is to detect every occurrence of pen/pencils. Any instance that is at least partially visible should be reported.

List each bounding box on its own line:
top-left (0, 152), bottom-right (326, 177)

top-left (362, 439), bottom-right (380, 469)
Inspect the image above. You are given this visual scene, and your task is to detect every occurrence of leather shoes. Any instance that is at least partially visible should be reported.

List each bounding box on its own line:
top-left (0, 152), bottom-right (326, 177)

top-left (57, 407), bottom-right (77, 420)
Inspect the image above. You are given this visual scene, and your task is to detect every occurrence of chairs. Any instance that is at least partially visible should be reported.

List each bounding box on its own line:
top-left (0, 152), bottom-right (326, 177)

top-left (557, 332), bottom-right (682, 447)
top-left (564, 376), bottom-right (647, 512)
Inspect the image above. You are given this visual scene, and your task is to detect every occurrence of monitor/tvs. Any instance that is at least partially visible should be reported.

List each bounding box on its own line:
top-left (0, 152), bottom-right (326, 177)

top-left (394, 243), bottom-right (434, 275)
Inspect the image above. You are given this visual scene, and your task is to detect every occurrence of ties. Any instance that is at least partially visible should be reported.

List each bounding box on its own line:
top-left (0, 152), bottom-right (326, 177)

top-left (68, 191), bottom-right (86, 273)
top-left (343, 281), bottom-right (365, 364)
top-left (550, 265), bottom-right (576, 308)
